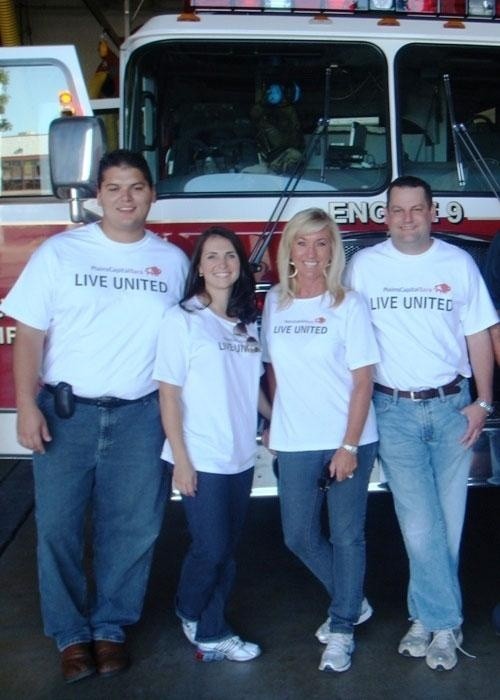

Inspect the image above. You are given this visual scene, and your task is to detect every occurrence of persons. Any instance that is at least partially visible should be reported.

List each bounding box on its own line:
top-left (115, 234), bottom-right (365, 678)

top-left (341, 176), bottom-right (500, 673)
top-left (481, 229), bottom-right (500, 365)
top-left (1, 149), bottom-right (190, 684)
top-left (152, 226), bottom-right (271, 663)
top-left (259, 208), bottom-right (381, 671)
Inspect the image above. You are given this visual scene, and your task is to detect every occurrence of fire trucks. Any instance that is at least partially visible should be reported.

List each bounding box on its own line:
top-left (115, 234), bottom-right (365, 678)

top-left (1, 1), bottom-right (500, 502)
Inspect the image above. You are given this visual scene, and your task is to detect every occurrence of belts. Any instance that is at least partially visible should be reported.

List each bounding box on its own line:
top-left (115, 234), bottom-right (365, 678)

top-left (43, 386), bottom-right (142, 405)
top-left (374, 375), bottom-right (463, 403)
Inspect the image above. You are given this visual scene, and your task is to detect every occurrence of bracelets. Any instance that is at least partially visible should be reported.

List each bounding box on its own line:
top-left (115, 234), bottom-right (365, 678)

top-left (476, 399), bottom-right (495, 414)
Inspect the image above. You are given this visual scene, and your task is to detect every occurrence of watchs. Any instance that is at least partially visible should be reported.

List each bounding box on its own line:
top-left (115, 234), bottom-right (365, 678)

top-left (340, 444), bottom-right (358, 456)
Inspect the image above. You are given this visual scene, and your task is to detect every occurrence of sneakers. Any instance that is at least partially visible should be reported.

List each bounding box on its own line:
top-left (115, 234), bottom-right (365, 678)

top-left (427, 627), bottom-right (462, 672)
top-left (397, 618), bottom-right (434, 658)
top-left (193, 636), bottom-right (263, 663)
top-left (177, 617), bottom-right (196, 644)
top-left (316, 596), bottom-right (373, 643)
top-left (317, 633), bottom-right (354, 672)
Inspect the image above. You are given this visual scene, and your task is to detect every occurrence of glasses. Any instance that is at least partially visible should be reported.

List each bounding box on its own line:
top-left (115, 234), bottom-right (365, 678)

top-left (317, 462), bottom-right (336, 491)
top-left (235, 324), bottom-right (257, 347)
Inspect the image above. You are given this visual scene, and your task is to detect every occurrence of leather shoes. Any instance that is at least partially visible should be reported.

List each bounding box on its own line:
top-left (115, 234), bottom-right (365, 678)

top-left (94, 640), bottom-right (128, 679)
top-left (61, 642), bottom-right (94, 684)
